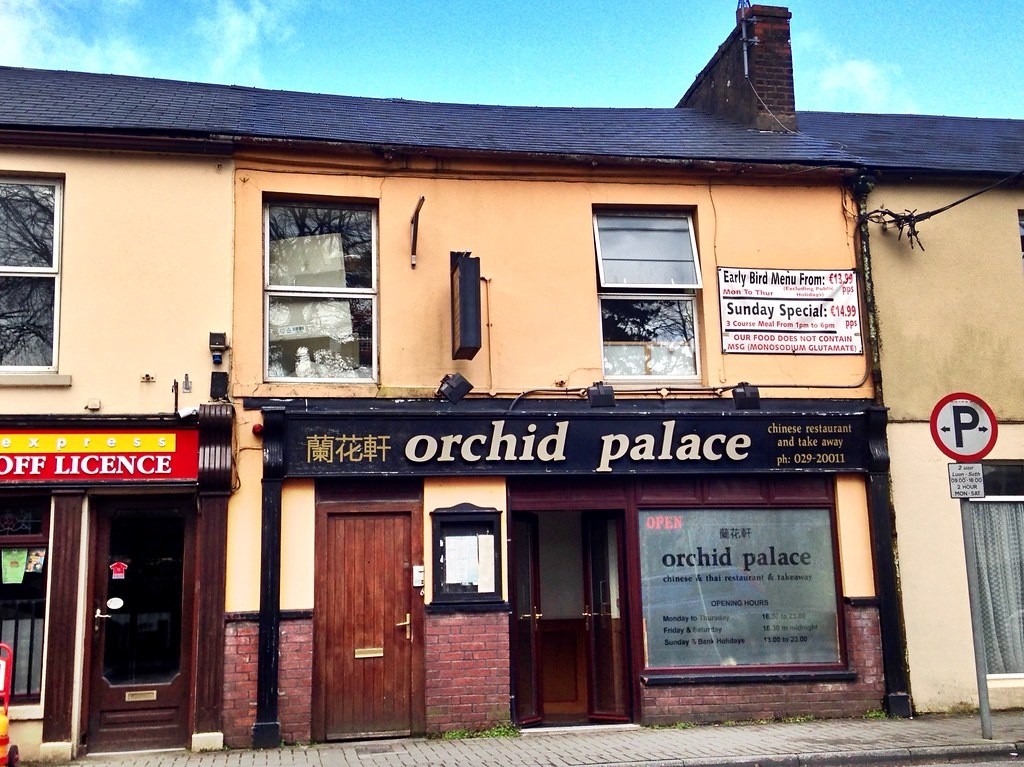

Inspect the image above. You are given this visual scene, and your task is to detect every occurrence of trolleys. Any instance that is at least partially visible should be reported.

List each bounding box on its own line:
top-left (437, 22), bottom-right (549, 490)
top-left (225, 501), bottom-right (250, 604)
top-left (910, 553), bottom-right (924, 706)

top-left (0, 642), bottom-right (19, 767)
